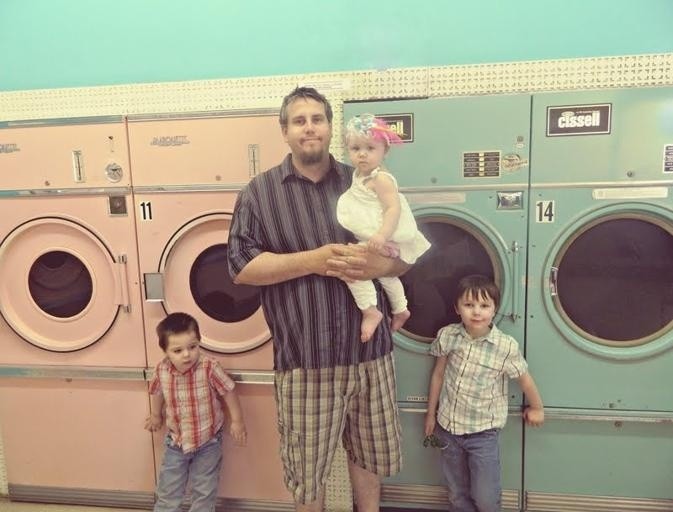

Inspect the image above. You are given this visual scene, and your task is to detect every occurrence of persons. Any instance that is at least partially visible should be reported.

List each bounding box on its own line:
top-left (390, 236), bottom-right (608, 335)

top-left (336, 108), bottom-right (431, 347)
top-left (225, 85), bottom-right (414, 511)
top-left (422, 272), bottom-right (545, 510)
top-left (143, 311), bottom-right (250, 511)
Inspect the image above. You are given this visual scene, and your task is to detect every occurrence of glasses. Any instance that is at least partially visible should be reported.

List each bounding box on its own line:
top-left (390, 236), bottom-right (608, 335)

top-left (424, 435), bottom-right (449, 449)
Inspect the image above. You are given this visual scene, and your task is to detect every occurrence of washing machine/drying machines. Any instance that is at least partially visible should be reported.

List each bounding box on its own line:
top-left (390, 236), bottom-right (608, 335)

top-left (123, 100), bottom-right (346, 509)
top-left (339, 91), bottom-right (534, 509)
top-left (527, 92), bottom-right (672, 511)
top-left (0, 109), bottom-right (157, 512)
